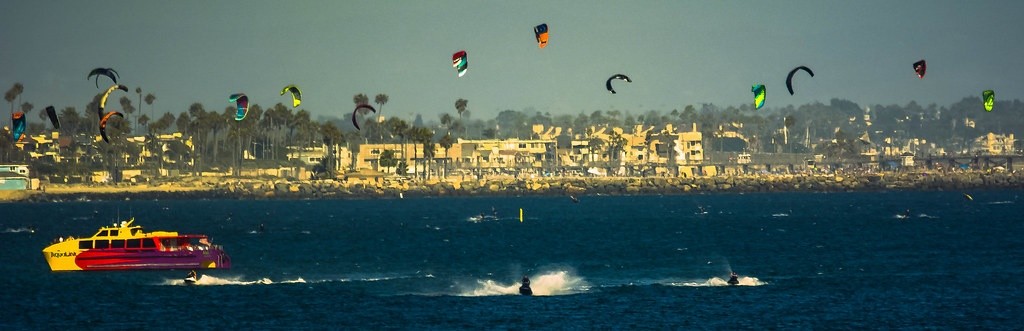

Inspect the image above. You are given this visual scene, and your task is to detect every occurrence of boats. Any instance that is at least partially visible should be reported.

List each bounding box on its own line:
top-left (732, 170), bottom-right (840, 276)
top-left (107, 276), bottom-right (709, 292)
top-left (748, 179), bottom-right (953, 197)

top-left (42, 216), bottom-right (232, 273)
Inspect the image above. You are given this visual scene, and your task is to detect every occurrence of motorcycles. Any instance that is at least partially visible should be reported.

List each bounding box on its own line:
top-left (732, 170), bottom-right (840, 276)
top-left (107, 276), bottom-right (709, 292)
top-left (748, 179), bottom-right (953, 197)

top-left (185, 274), bottom-right (196, 283)
top-left (728, 276), bottom-right (740, 285)
top-left (520, 284), bottom-right (534, 296)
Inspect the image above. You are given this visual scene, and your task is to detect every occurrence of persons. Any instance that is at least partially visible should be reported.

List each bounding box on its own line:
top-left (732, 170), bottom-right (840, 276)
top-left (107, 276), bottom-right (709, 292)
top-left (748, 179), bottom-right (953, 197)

top-left (191, 270), bottom-right (196, 280)
top-left (522, 275), bottom-right (530, 286)
